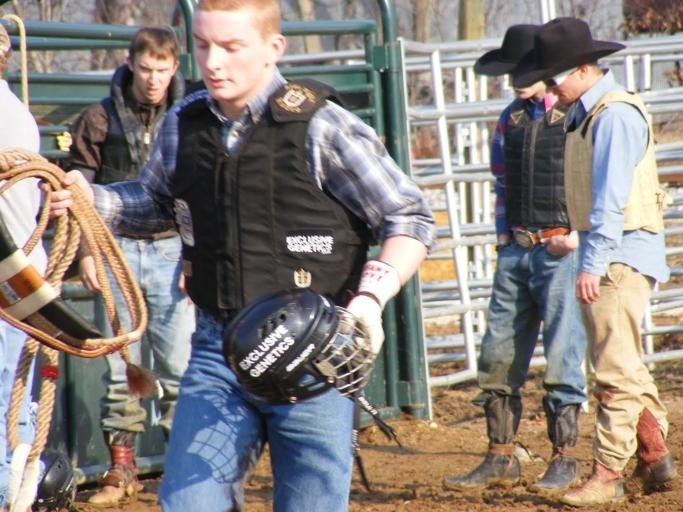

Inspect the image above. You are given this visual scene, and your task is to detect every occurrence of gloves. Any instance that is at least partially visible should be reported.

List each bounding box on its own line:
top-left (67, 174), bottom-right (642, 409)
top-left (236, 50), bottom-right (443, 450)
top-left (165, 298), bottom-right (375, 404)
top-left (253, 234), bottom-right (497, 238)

top-left (340, 259), bottom-right (401, 365)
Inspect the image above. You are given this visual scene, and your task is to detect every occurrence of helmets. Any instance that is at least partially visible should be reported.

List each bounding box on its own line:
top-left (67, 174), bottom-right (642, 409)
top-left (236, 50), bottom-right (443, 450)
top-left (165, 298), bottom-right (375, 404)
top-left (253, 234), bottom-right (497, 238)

top-left (31, 449), bottom-right (78, 512)
top-left (222, 287), bottom-right (376, 405)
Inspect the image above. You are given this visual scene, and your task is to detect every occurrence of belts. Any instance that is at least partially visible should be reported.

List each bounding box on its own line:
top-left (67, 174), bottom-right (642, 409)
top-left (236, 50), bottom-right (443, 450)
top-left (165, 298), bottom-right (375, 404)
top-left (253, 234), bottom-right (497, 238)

top-left (512, 226), bottom-right (570, 250)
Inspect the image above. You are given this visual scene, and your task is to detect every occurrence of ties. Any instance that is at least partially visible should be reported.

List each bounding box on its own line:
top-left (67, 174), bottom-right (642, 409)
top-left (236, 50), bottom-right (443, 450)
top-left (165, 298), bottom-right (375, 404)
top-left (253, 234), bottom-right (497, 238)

top-left (575, 97), bottom-right (587, 129)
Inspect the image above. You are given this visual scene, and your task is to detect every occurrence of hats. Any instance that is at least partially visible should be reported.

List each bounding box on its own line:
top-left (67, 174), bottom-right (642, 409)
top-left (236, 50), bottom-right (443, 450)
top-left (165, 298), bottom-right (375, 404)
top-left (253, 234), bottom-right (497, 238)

top-left (513, 17), bottom-right (626, 88)
top-left (474, 24), bottom-right (541, 76)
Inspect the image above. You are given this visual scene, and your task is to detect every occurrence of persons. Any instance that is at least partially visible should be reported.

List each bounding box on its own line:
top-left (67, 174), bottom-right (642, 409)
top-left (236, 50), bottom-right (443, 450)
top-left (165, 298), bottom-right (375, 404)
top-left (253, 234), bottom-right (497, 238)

top-left (67, 26), bottom-right (199, 506)
top-left (442, 24), bottom-right (592, 494)
top-left (37, 0), bottom-right (438, 512)
top-left (1, 24), bottom-right (51, 512)
top-left (510, 16), bottom-right (678, 506)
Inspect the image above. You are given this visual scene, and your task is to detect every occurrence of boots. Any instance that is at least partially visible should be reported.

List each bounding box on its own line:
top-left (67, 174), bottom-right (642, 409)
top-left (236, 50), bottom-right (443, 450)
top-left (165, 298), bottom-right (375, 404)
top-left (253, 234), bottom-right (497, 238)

top-left (623, 406), bottom-right (677, 499)
top-left (442, 395), bottom-right (521, 492)
top-left (529, 403), bottom-right (581, 492)
top-left (559, 458), bottom-right (625, 507)
top-left (88, 428), bottom-right (144, 508)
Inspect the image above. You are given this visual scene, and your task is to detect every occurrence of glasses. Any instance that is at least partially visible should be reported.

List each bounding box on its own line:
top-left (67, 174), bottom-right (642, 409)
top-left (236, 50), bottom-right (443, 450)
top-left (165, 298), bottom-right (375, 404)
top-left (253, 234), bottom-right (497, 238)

top-left (542, 65), bottom-right (581, 89)
